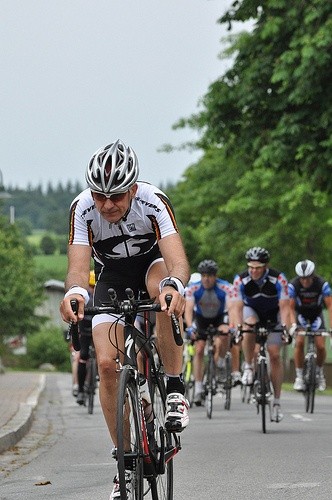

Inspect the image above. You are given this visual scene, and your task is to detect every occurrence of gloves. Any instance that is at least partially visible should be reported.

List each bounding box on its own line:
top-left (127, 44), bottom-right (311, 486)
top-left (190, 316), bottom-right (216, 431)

top-left (232, 334), bottom-right (244, 345)
top-left (281, 335), bottom-right (292, 345)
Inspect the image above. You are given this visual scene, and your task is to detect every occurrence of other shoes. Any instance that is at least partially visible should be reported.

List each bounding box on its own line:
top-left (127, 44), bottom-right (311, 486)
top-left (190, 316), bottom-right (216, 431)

top-left (76, 393), bottom-right (84, 404)
top-left (73, 388), bottom-right (78, 396)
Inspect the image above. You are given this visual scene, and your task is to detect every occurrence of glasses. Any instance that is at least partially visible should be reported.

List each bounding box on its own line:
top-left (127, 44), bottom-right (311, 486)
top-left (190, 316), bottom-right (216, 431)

top-left (201, 273), bottom-right (215, 278)
top-left (91, 190), bottom-right (128, 203)
top-left (246, 263), bottom-right (266, 271)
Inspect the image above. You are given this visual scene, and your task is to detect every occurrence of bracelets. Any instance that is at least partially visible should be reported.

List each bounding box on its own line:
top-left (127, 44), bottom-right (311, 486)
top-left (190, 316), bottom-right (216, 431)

top-left (63, 286), bottom-right (90, 307)
top-left (159, 277), bottom-right (185, 297)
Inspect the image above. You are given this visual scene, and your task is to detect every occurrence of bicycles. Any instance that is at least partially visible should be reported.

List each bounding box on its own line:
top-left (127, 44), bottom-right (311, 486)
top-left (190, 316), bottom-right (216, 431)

top-left (70, 288), bottom-right (182, 500)
top-left (183, 339), bottom-right (252, 411)
top-left (295, 324), bottom-right (332, 414)
top-left (190, 324), bottom-right (229, 419)
top-left (234, 321), bottom-right (292, 435)
top-left (77, 326), bottom-right (99, 415)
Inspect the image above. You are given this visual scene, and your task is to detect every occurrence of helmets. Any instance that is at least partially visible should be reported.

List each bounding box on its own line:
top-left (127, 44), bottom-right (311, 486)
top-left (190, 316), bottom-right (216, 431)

top-left (89, 270), bottom-right (95, 286)
top-left (85, 139), bottom-right (139, 195)
top-left (294, 259), bottom-right (315, 279)
top-left (197, 259), bottom-right (218, 273)
top-left (245, 247), bottom-right (270, 263)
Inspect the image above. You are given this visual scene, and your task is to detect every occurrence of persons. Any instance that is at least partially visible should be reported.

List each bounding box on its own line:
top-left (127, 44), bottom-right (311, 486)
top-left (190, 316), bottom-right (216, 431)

top-left (60, 138), bottom-right (191, 500)
top-left (69, 247), bottom-right (332, 423)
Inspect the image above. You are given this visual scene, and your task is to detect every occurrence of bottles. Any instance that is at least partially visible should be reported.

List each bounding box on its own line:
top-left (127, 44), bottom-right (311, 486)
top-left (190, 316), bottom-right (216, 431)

top-left (140, 378), bottom-right (154, 424)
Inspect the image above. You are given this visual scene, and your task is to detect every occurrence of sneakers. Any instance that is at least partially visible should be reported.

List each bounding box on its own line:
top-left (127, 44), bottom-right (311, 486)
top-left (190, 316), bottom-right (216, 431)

top-left (217, 366), bottom-right (227, 383)
top-left (230, 371), bottom-right (242, 387)
top-left (164, 393), bottom-right (190, 427)
top-left (272, 403), bottom-right (283, 422)
top-left (242, 368), bottom-right (253, 386)
top-left (109, 470), bottom-right (136, 500)
top-left (316, 372), bottom-right (326, 391)
top-left (194, 381), bottom-right (205, 393)
top-left (293, 377), bottom-right (306, 392)
